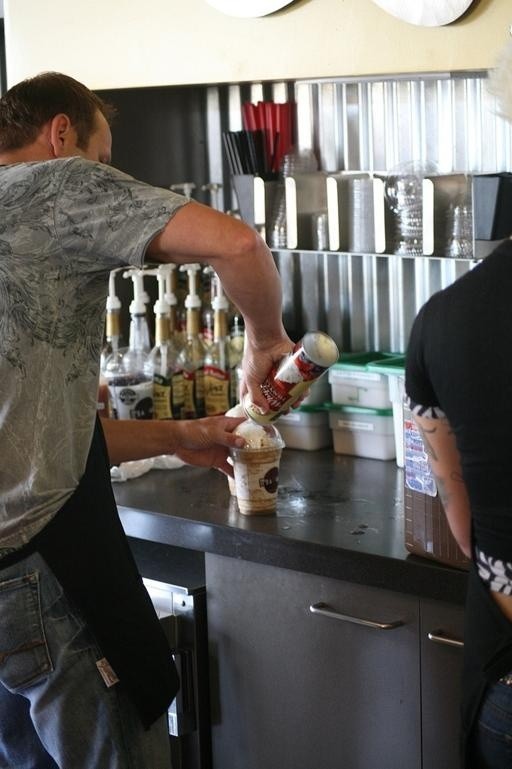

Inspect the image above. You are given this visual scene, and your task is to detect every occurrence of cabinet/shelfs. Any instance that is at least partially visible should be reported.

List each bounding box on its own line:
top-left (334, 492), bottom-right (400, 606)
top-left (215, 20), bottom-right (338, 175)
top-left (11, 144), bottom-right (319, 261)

top-left (203, 548), bottom-right (485, 766)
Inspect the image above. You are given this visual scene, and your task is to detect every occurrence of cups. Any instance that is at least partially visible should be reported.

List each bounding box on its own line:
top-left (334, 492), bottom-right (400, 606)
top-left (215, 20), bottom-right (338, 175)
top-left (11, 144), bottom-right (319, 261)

top-left (231, 438), bottom-right (286, 516)
top-left (266, 150), bottom-right (473, 259)
top-left (227, 455), bottom-right (235, 496)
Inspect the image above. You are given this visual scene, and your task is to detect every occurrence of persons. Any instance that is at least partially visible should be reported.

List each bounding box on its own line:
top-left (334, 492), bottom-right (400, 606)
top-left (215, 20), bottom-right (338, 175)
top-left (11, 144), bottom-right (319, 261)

top-left (0, 71), bottom-right (295, 769)
top-left (403, 238), bottom-right (512, 769)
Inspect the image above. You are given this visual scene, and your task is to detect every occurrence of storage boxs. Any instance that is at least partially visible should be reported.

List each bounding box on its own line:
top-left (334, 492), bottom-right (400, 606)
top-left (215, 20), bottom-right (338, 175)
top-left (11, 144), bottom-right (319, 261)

top-left (268, 411), bottom-right (333, 453)
top-left (302, 370), bottom-right (332, 407)
top-left (367, 353), bottom-right (408, 471)
top-left (327, 403), bottom-right (398, 464)
top-left (326, 347), bottom-right (405, 407)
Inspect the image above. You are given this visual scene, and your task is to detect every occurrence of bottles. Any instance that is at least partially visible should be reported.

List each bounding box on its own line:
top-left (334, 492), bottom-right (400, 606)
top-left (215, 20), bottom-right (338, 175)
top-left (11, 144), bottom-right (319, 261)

top-left (238, 332), bottom-right (339, 428)
top-left (94, 252), bottom-right (244, 420)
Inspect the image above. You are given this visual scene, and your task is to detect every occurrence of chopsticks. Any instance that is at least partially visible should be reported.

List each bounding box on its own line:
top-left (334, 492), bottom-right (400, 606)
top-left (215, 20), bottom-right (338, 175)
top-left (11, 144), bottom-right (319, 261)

top-left (219, 101), bottom-right (293, 175)
